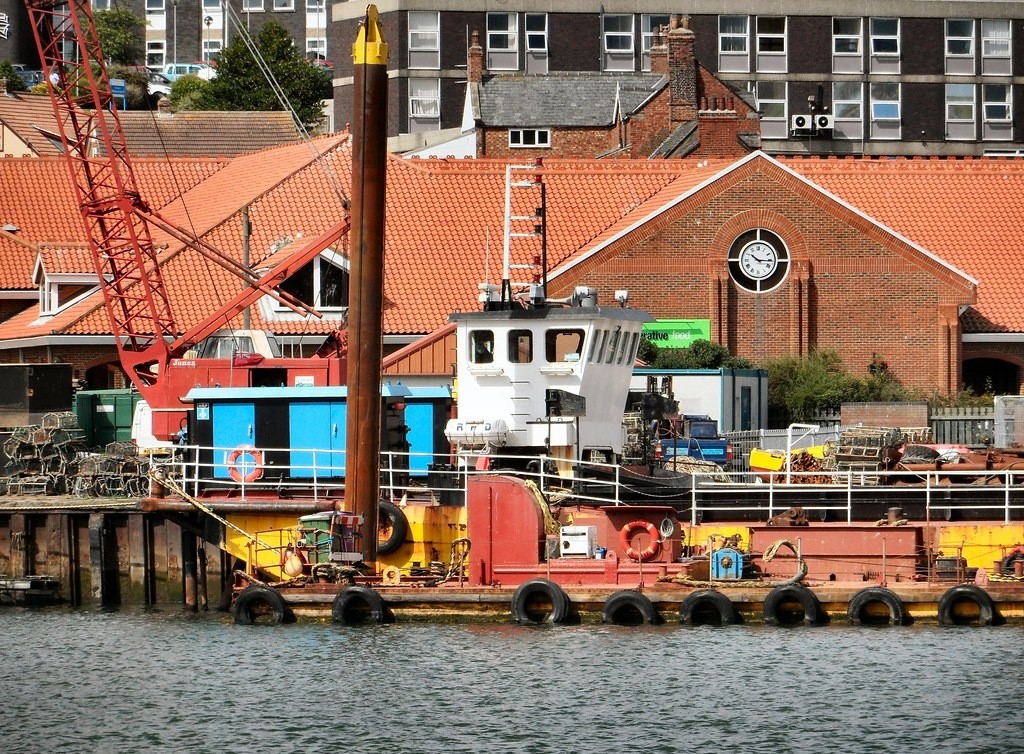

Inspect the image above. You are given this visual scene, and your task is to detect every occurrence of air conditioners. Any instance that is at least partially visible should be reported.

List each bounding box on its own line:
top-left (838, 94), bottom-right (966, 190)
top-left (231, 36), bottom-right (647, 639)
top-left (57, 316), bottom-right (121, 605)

top-left (792, 114), bottom-right (812, 129)
top-left (814, 114), bottom-right (834, 129)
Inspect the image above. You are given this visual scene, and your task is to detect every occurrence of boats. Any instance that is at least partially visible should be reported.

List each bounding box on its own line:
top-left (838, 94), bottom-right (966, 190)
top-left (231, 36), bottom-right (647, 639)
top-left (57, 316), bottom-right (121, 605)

top-left (229, 473), bottom-right (1024, 628)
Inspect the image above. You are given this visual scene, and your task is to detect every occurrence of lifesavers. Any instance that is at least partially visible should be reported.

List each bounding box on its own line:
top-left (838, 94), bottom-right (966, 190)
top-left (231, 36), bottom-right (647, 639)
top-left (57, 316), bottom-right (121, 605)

top-left (762, 582), bottom-right (822, 627)
top-left (619, 519), bottom-right (660, 560)
top-left (231, 583), bottom-right (289, 628)
top-left (846, 585), bottom-right (907, 627)
top-left (601, 588), bottom-right (657, 627)
top-left (677, 588), bottom-right (736, 628)
top-left (937, 583), bottom-right (998, 628)
top-left (376, 499), bottom-right (410, 557)
top-left (509, 577), bottom-right (571, 628)
top-left (226, 444), bottom-right (267, 483)
top-left (331, 585), bottom-right (389, 627)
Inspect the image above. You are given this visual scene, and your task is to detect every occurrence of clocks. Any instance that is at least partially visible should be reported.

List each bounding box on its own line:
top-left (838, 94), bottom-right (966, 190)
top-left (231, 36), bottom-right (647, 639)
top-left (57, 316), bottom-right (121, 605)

top-left (739, 239), bottom-right (779, 281)
